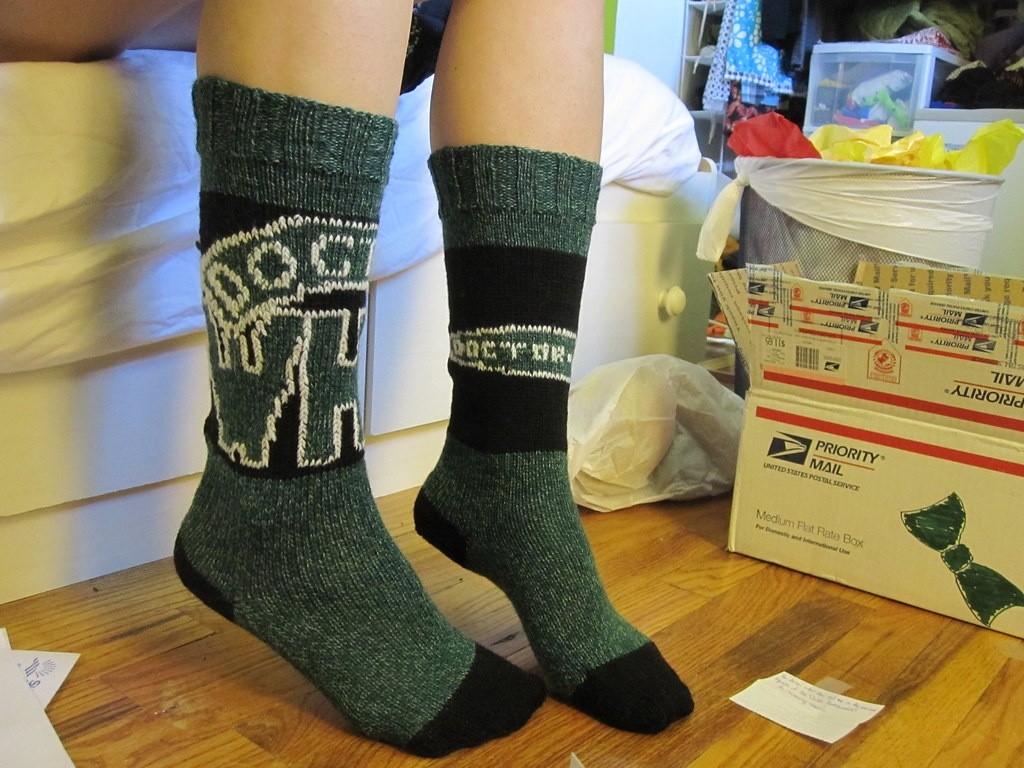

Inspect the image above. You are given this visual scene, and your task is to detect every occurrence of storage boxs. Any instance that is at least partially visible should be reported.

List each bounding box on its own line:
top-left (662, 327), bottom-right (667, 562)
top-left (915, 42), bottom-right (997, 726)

top-left (802, 41), bottom-right (976, 137)
top-left (705, 259), bottom-right (1024, 640)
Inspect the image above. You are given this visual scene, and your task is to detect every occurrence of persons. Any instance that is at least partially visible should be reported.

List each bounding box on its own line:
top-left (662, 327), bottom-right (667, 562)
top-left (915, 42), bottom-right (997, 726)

top-left (0, 1), bottom-right (693, 759)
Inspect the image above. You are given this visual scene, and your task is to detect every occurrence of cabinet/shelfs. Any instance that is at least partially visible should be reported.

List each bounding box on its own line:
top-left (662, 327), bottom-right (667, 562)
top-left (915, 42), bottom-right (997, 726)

top-left (676, 0), bottom-right (738, 183)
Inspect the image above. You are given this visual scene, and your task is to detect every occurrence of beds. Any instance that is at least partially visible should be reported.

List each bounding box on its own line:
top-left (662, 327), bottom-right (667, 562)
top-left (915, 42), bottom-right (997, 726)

top-left (1, 45), bottom-right (726, 600)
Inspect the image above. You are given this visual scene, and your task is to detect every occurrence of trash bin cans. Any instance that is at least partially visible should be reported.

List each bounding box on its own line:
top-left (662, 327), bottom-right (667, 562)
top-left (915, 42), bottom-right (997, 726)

top-left (732, 157), bottom-right (1006, 399)
